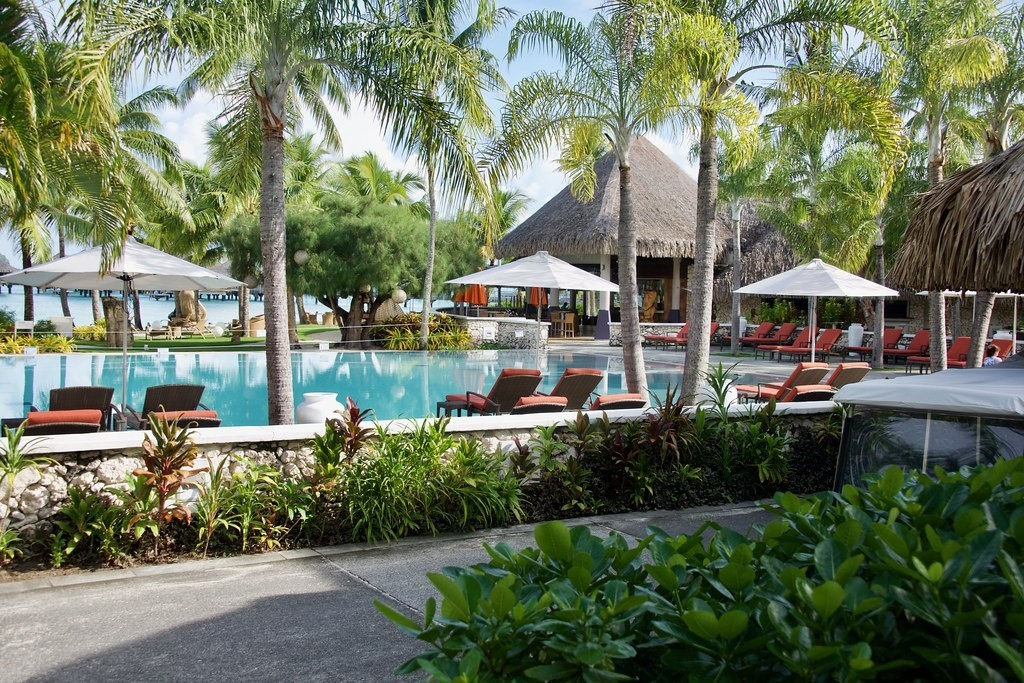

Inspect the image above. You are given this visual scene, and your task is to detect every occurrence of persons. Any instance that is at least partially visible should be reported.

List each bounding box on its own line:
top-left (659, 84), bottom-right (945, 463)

top-left (983, 344), bottom-right (1003, 367)
top-left (561, 302), bottom-right (568, 309)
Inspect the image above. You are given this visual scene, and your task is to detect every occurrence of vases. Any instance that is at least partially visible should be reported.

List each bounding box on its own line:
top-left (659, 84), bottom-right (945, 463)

top-left (296, 392), bottom-right (343, 424)
top-left (848, 323), bottom-right (864, 356)
top-left (993, 330), bottom-right (1013, 358)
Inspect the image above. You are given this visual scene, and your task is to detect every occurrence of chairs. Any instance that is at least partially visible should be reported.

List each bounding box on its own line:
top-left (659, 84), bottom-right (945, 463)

top-left (1, 384), bottom-right (222, 437)
top-left (145, 319), bottom-right (216, 341)
top-left (735, 361), bottom-right (873, 403)
top-left (479, 310), bottom-right (575, 338)
top-left (642, 320), bottom-right (842, 363)
top-left (435, 368), bottom-right (646, 419)
top-left (840, 329), bottom-right (1013, 375)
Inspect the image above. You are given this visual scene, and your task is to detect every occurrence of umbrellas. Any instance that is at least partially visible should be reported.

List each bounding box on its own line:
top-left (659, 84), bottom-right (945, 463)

top-left (0, 234), bottom-right (249, 432)
top-left (442, 250), bottom-right (620, 395)
top-left (733, 257), bottom-right (901, 363)
top-left (530, 287), bottom-right (547, 323)
top-left (451, 284), bottom-right (488, 317)
top-left (916, 289), bottom-right (1024, 355)
